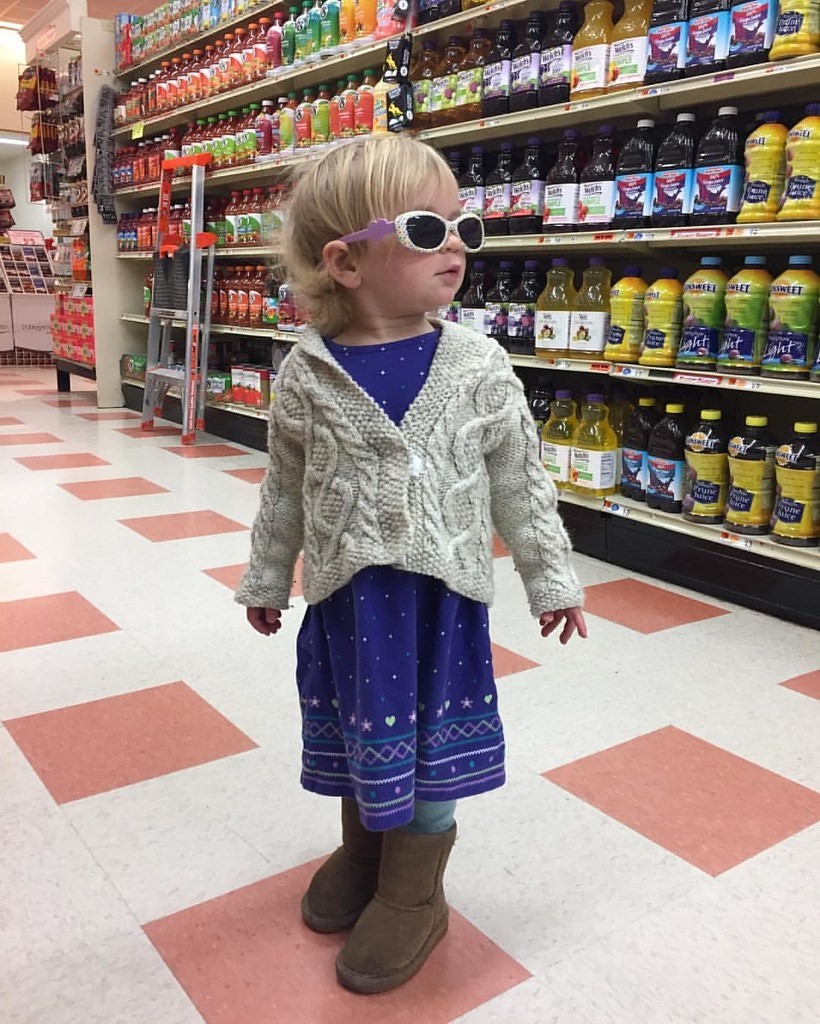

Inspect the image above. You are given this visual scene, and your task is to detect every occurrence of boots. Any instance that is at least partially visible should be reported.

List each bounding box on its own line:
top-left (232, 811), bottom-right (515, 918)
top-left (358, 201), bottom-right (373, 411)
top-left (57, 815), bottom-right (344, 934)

top-left (299, 797), bottom-right (384, 933)
top-left (335, 823), bottom-right (459, 994)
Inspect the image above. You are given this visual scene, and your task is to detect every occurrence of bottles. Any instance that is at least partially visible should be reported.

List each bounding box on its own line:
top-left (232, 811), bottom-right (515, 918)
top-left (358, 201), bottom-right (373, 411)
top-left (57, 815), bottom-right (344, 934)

top-left (109, 0), bottom-right (820, 547)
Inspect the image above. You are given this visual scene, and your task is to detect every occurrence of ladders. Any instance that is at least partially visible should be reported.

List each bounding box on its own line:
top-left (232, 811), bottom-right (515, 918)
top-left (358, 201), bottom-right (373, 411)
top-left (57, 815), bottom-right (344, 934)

top-left (141, 152), bottom-right (218, 446)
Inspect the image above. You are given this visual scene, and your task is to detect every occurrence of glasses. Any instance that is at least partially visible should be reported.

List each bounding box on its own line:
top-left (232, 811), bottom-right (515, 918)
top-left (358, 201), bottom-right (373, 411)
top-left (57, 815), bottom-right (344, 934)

top-left (338, 209), bottom-right (485, 254)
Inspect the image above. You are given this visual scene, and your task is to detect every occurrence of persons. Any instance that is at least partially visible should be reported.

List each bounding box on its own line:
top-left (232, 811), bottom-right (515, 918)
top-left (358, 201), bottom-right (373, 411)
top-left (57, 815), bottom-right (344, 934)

top-left (234, 129), bottom-right (588, 997)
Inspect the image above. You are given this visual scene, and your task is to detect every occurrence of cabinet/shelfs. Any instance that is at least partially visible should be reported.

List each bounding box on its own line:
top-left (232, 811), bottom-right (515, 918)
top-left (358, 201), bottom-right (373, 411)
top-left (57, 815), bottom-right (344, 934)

top-left (42, 86), bottom-right (97, 394)
top-left (115, 0), bottom-right (820, 632)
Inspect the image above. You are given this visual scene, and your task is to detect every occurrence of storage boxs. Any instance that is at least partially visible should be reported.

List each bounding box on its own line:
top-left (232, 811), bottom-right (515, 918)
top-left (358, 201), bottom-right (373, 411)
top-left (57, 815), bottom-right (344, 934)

top-left (48, 292), bottom-right (96, 366)
